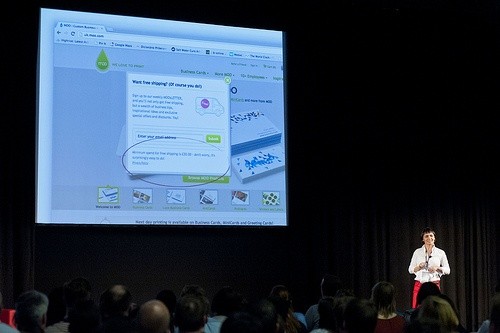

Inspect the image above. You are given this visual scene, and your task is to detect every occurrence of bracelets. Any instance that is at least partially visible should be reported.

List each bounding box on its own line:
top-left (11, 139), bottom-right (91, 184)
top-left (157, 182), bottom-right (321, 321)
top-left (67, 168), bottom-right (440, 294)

top-left (437, 267), bottom-right (439, 271)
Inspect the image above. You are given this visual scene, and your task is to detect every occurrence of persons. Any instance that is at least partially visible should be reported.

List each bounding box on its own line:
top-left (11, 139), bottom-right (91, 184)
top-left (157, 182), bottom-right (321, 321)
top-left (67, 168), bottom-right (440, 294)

top-left (407, 226), bottom-right (451, 312)
top-left (0, 270), bottom-right (500, 333)
